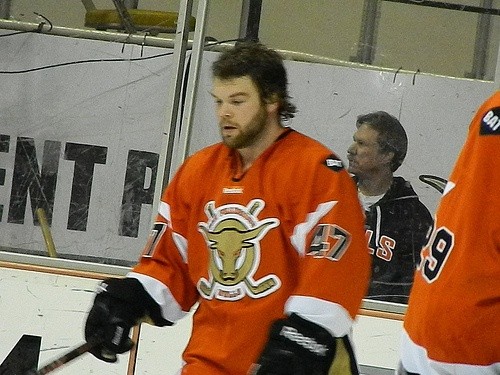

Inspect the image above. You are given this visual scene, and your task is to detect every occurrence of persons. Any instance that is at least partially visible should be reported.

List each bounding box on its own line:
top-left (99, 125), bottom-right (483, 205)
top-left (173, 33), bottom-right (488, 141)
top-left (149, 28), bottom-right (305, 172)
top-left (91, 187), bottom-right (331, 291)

top-left (344, 111), bottom-right (435, 306)
top-left (85, 37), bottom-right (373, 374)
top-left (396, 89), bottom-right (500, 375)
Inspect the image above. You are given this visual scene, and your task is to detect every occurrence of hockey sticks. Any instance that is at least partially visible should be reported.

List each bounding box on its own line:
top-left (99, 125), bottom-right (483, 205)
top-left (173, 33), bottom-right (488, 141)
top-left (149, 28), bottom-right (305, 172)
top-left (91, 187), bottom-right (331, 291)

top-left (0, 333), bottom-right (100, 375)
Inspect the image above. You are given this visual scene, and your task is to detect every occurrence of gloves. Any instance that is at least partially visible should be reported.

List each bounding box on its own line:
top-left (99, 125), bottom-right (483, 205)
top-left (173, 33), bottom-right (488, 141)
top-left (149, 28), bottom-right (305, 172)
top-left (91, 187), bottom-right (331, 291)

top-left (252, 313), bottom-right (337, 375)
top-left (85, 277), bottom-right (175, 364)
